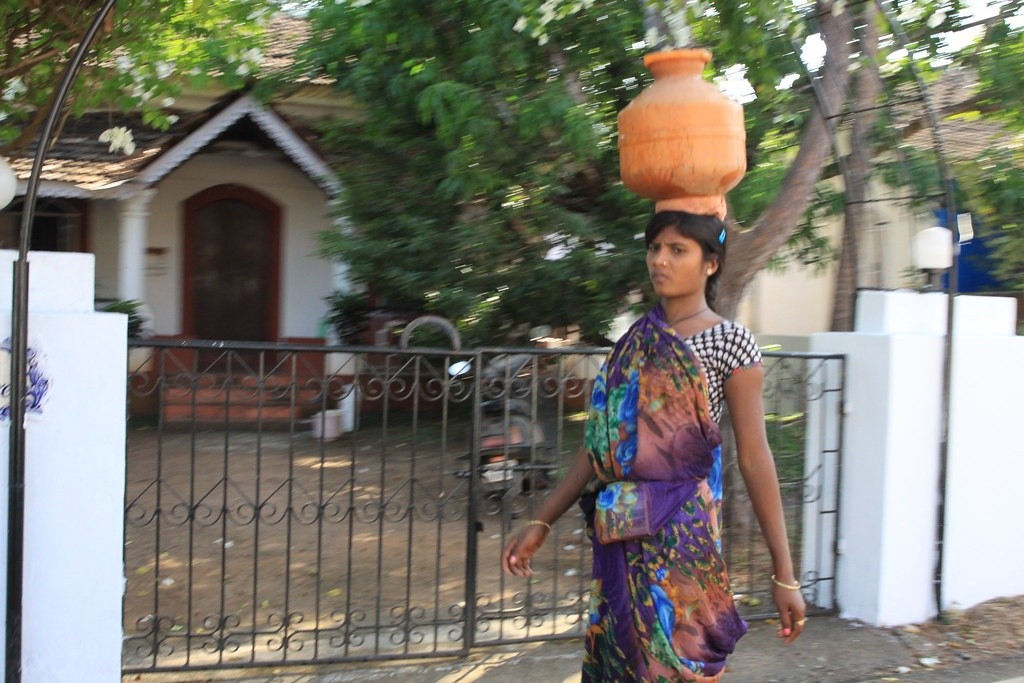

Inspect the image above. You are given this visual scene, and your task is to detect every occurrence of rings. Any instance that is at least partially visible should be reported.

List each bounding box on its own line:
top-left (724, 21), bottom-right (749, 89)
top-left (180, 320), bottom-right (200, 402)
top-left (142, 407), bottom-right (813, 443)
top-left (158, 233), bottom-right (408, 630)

top-left (796, 617), bottom-right (808, 626)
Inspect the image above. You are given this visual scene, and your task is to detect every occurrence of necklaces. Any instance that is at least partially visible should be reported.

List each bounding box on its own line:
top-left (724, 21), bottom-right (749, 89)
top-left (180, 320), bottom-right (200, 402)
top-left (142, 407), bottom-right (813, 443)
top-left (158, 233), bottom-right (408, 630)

top-left (666, 305), bottom-right (708, 327)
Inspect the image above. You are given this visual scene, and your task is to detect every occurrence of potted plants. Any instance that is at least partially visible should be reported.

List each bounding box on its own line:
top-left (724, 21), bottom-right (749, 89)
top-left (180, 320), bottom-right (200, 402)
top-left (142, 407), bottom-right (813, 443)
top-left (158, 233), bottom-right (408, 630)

top-left (312, 395), bottom-right (344, 444)
top-left (321, 289), bottom-right (374, 377)
top-left (100, 299), bottom-right (157, 374)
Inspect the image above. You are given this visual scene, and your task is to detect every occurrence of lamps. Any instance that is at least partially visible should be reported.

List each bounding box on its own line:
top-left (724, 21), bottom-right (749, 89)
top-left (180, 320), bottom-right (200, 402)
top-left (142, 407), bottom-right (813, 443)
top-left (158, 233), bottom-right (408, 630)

top-left (915, 225), bottom-right (954, 293)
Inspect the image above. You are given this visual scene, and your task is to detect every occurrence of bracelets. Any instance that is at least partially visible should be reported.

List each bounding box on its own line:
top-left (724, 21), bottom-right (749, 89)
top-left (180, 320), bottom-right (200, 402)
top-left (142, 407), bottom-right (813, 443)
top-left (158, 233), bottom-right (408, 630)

top-left (526, 521), bottom-right (551, 533)
top-left (771, 575), bottom-right (801, 589)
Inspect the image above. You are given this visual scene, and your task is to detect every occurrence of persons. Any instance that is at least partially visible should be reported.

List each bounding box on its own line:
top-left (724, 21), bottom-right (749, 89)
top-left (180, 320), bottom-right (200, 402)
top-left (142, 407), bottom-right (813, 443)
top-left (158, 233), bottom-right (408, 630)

top-left (501, 210), bottom-right (808, 683)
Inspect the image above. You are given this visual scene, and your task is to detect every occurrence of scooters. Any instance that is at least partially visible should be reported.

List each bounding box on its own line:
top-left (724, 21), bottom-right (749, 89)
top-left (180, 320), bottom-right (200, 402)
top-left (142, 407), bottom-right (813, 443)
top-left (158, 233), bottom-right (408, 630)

top-left (447, 322), bottom-right (555, 516)
